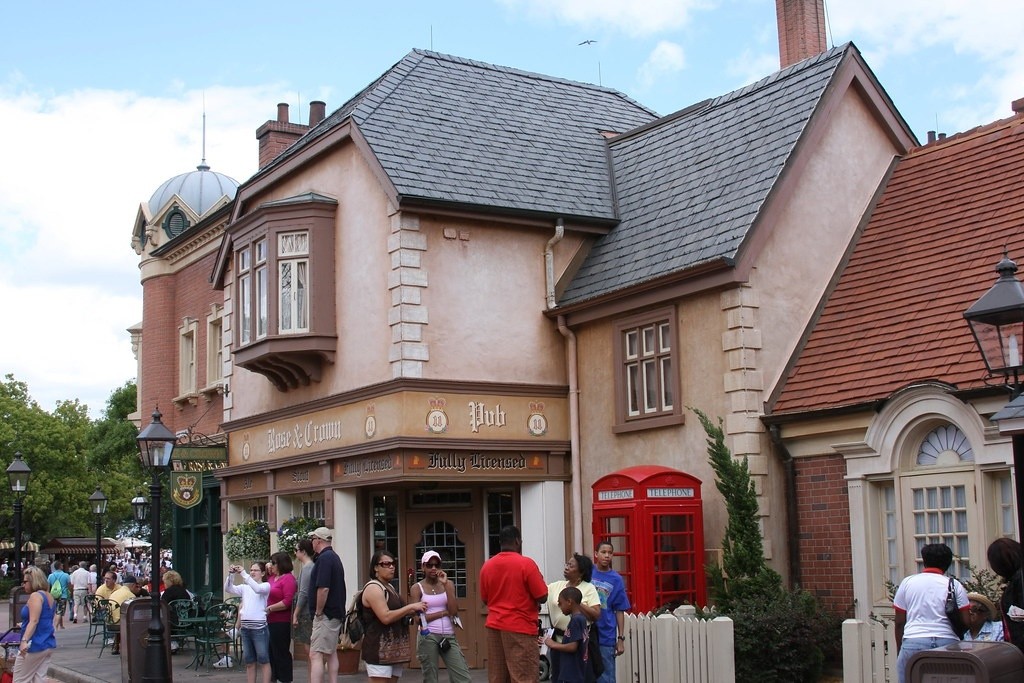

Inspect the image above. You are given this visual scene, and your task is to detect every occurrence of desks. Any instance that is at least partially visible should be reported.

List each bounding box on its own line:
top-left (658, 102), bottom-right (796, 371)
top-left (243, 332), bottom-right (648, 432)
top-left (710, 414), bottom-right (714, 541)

top-left (178, 616), bottom-right (222, 673)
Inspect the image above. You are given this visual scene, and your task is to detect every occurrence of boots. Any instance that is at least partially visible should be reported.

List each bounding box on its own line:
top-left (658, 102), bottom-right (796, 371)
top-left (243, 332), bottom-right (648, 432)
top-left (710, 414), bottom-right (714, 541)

top-left (111, 634), bottom-right (121, 655)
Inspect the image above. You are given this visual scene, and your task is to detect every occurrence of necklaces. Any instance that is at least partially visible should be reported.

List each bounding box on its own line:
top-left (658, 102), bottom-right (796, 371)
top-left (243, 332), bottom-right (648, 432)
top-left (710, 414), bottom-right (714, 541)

top-left (428, 580), bottom-right (439, 595)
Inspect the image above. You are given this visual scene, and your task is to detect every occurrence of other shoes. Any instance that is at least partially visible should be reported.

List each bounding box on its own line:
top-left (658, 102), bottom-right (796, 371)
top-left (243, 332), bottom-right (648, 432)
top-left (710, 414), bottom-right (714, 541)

top-left (73, 619), bottom-right (77, 623)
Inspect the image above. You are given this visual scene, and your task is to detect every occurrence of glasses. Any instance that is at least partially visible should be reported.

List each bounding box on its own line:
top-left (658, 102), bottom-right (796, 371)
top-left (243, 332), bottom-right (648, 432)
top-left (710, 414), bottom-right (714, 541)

top-left (21, 580), bottom-right (29, 585)
top-left (968, 606), bottom-right (986, 613)
top-left (250, 569), bottom-right (261, 572)
top-left (425, 562), bottom-right (441, 568)
top-left (312, 538), bottom-right (321, 541)
top-left (271, 561), bottom-right (278, 565)
top-left (376, 561), bottom-right (394, 568)
top-left (295, 549), bottom-right (304, 552)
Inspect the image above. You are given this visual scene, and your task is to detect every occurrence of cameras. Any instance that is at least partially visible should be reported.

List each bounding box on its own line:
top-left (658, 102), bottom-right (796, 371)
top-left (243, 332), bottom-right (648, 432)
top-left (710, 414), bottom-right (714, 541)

top-left (233, 566), bottom-right (238, 572)
top-left (403, 616), bottom-right (415, 626)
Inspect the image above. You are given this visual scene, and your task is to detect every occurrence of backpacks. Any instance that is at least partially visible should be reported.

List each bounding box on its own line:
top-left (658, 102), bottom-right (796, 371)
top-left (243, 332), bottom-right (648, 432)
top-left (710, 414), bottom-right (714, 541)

top-left (50, 573), bottom-right (64, 600)
top-left (339, 581), bottom-right (390, 650)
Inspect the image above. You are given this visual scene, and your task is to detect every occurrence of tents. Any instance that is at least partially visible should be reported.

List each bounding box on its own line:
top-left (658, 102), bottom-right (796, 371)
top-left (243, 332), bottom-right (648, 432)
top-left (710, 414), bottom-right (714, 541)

top-left (106, 537), bottom-right (153, 554)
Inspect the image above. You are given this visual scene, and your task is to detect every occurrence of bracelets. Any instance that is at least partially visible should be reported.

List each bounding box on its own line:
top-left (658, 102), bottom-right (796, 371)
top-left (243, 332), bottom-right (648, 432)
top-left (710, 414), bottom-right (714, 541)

top-left (268, 606), bottom-right (272, 613)
top-left (315, 612), bottom-right (324, 617)
top-left (20, 638), bottom-right (28, 643)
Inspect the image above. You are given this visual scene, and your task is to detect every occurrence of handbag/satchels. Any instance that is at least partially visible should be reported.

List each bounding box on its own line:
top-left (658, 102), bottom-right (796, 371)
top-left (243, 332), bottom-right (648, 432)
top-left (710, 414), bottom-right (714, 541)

top-left (945, 578), bottom-right (968, 640)
top-left (438, 637), bottom-right (450, 652)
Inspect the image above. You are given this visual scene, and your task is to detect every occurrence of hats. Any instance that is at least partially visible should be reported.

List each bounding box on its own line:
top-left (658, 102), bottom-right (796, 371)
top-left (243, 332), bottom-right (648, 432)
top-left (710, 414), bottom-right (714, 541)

top-left (968, 591), bottom-right (998, 620)
top-left (308, 527), bottom-right (332, 541)
top-left (123, 575), bottom-right (139, 584)
top-left (421, 550), bottom-right (441, 565)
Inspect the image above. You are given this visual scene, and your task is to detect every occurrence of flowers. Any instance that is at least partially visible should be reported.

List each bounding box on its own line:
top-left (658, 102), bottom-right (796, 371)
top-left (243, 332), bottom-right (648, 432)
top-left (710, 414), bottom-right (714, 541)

top-left (225, 518), bottom-right (272, 565)
top-left (276, 515), bottom-right (326, 562)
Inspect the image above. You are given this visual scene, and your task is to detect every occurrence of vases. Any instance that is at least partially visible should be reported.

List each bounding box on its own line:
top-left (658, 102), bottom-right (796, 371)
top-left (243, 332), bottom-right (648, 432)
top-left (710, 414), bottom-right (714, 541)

top-left (335, 648), bottom-right (361, 675)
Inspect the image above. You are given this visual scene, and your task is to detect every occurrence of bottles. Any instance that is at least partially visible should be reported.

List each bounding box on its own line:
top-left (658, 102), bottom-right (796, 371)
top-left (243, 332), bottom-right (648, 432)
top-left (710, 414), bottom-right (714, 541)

top-left (416, 610), bottom-right (431, 637)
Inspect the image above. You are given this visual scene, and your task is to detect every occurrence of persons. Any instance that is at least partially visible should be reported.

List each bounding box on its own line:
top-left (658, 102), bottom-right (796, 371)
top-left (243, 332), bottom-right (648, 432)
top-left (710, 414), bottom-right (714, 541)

top-left (410, 550), bottom-right (473, 683)
top-left (356, 550), bottom-right (428, 683)
top-left (539, 586), bottom-right (588, 683)
top-left (891, 538), bottom-right (1024, 683)
top-left (547, 552), bottom-right (601, 683)
top-left (12, 565), bottom-right (58, 683)
top-left (0, 548), bottom-right (199, 654)
top-left (479, 525), bottom-right (548, 683)
top-left (224, 551), bottom-right (298, 683)
top-left (292, 539), bottom-right (316, 683)
top-left (308, 527), bottom-right (346, 683)
top-left (589, 540), bottom-right (632, 683)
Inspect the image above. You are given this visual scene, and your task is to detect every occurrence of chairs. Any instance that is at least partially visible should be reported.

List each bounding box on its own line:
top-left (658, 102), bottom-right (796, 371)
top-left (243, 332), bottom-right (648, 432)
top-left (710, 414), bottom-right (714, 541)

top-left (84, 594), bottom-right (122, 659)
top-left (168, 592), bottom-right (244, 672)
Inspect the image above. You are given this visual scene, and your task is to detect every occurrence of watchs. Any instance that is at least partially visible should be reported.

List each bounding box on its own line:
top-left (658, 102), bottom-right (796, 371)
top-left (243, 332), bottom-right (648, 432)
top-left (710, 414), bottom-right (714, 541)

top-left (617, 634), bottom-right (625, 640)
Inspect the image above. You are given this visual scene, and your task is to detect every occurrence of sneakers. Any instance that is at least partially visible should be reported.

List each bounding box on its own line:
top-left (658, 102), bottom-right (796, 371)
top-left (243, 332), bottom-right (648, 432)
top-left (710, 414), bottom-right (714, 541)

top-left (213, 658), bottom-right (233, 667)
top-left (221, 628), bottom-right (239, 640)
